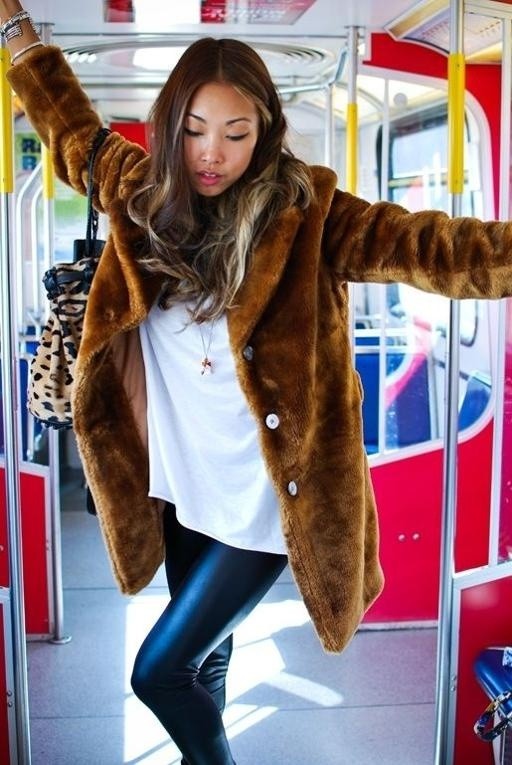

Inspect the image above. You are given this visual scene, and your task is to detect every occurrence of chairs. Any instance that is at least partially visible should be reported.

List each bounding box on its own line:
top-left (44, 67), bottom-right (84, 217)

top-left (2, 307), bottom-right (69, 647)
top-left (323, 308), bottom-right (510, 764)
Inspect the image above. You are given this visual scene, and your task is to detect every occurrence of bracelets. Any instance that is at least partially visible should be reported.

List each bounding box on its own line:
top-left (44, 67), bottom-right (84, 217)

top-left (2, 10), bottom-right (30, 43)
top-left (11, 40), bottom-right (44, 65)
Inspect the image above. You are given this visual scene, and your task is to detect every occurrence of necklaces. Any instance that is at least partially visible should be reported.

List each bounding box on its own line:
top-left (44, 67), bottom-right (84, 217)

top-left (182, 268), bottom-right (224, 381)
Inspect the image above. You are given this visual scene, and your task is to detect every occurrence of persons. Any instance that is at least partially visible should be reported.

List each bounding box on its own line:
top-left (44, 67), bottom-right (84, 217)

top-left (0, 0), bottom-right (511, 765)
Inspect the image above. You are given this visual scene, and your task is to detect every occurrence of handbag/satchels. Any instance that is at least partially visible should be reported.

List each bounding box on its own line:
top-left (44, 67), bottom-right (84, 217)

top-left (26, 257), bottom-right (100, 432)
